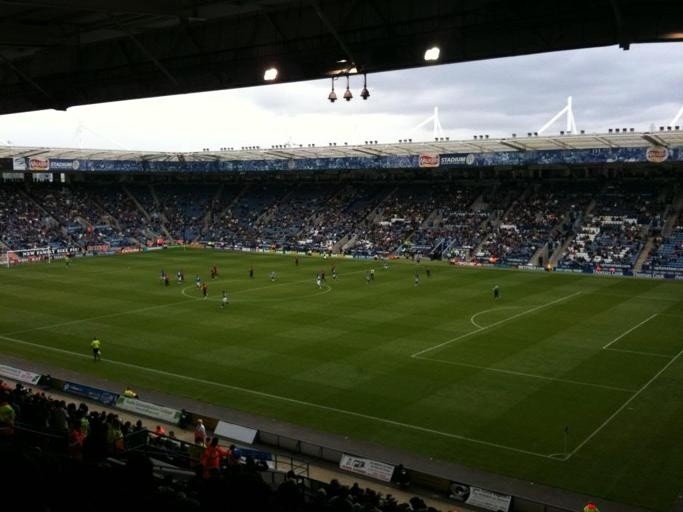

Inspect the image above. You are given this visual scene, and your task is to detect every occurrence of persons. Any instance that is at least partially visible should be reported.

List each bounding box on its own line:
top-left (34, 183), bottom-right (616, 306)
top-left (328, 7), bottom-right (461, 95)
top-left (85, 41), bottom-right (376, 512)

top-left (583, 501), bottom-right (600, 512)
top-left (201, 281), bottom-right (209, 298)
top-left (91, 337), bottom-right (101, 361)
top-left (221, 289), bottom-right (231, 307)
top-left (1, 371), bottom-right (440, 511)
top-left (1, 167), bottom-right (682, 290)
top-left (492, 284), bottom-right (502, 301)
